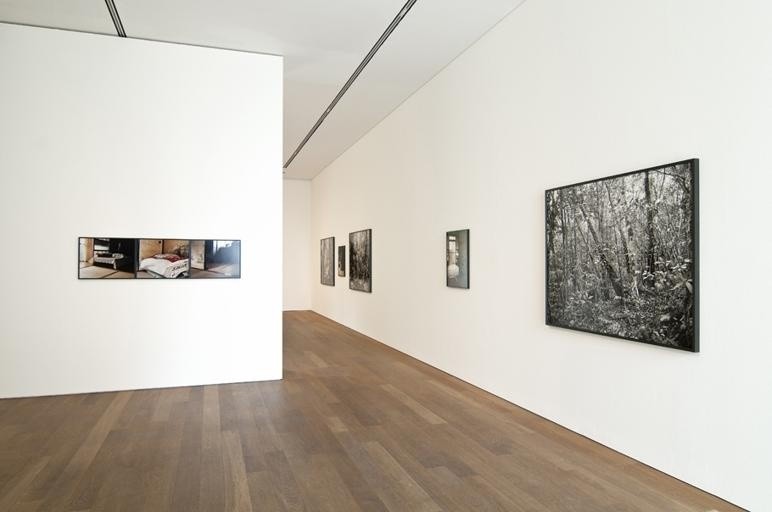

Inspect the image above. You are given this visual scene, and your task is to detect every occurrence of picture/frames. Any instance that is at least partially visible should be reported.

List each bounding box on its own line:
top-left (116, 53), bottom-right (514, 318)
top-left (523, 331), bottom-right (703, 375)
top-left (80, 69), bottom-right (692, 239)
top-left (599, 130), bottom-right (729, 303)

top-left (337, 246), bottom-right (345, 278)
top-left (445, 229), bottom-right (471, 290)
top-left (319, 236), bottom-right (336, 286)
top-left (348, 228), bottom-right (372, 293)
top-left (191, 239), bottom-right (243, 280)
top-left (136, 238), bottom-right (191, 280)
top-left (76, 236), bottom-right (135, 280)
top-left (542, 157), bottom-right (702, 354)
